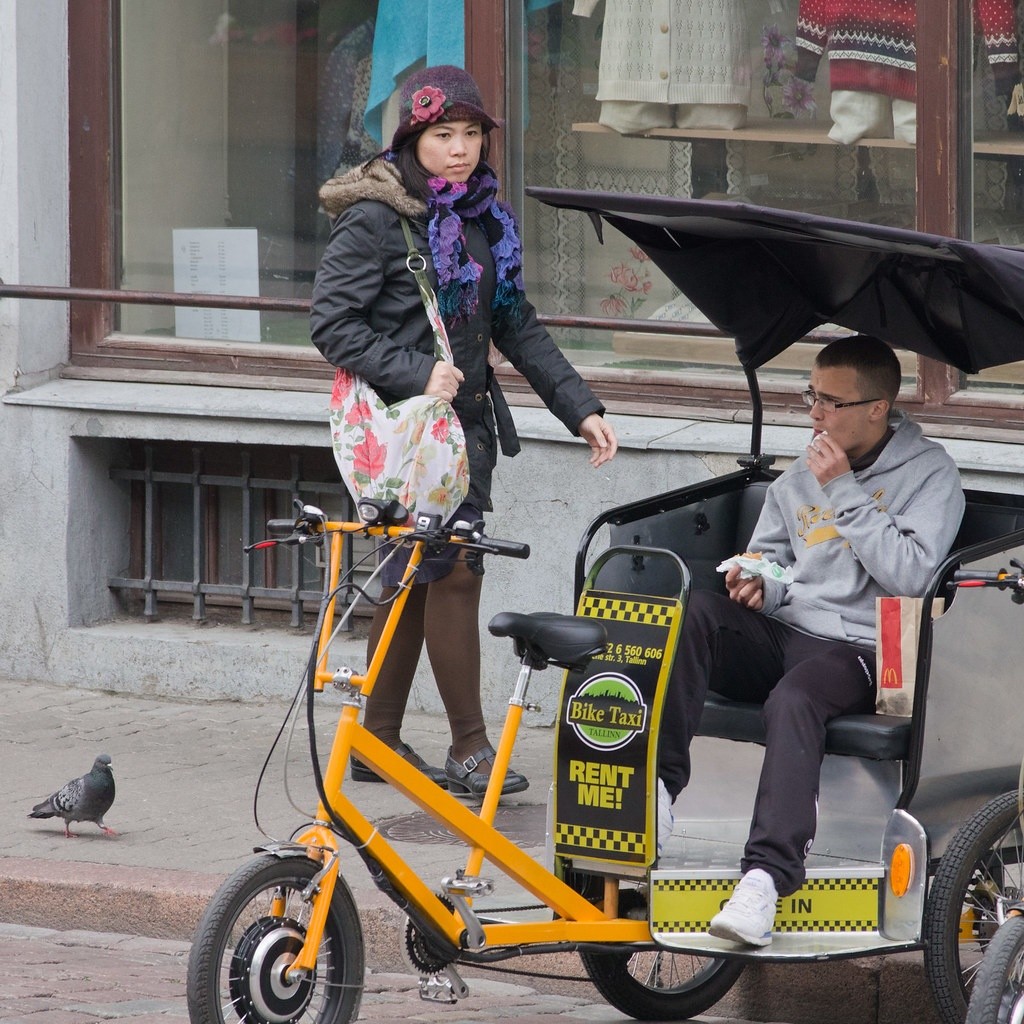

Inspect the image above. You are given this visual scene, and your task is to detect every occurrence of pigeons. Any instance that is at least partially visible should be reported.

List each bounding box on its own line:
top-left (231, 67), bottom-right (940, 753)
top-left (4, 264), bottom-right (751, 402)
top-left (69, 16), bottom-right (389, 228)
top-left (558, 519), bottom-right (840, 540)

top-left (26, 755), bottom-right (117, 839)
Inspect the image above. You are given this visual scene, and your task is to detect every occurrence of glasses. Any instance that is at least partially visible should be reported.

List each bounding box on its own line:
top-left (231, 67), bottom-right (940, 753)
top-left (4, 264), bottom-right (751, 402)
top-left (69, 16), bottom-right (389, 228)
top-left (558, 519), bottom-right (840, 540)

top-left (802, 390), bottom-right (885, 412)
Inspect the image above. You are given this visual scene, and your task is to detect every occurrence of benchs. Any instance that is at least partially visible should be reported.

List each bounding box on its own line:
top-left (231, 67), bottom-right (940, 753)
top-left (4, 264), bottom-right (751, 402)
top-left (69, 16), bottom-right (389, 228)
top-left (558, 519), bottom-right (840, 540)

top-left (569, 453), bottom-right (1024, 758)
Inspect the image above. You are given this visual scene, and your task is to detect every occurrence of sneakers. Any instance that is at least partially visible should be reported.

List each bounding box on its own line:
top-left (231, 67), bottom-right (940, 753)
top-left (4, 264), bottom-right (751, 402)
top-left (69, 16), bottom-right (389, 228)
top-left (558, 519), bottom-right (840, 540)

top-left (658, 777), bottom-right (676, 859)
top-left (708, 869), bottom-right (779, 946)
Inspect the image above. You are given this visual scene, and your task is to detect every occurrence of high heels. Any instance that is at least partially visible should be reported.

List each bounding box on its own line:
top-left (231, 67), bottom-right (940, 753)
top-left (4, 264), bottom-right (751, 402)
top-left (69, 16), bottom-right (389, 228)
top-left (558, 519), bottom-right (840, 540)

top-left (350, 744), bottom-right (445, 785)
top-left (446, 744), bottom-right (529, 796)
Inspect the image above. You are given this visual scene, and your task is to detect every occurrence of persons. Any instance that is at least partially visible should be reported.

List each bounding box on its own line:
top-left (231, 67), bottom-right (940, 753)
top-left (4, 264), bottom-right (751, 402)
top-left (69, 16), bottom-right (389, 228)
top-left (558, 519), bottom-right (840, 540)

top-left (656, 336), bottom-right (966, 946)
top-left (310, 65), bottom-right (618, 795)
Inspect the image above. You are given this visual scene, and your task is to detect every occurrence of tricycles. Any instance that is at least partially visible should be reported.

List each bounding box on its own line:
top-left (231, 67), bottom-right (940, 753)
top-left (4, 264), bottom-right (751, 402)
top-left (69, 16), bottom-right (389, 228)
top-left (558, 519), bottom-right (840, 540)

top-left (184, 440), bottom-right (1024, 1024)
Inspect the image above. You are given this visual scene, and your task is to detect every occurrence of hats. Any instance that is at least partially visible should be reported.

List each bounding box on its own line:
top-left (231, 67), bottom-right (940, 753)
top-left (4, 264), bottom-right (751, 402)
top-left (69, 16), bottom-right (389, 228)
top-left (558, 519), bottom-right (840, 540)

top-left (391, 66), bottom-right (503, 155)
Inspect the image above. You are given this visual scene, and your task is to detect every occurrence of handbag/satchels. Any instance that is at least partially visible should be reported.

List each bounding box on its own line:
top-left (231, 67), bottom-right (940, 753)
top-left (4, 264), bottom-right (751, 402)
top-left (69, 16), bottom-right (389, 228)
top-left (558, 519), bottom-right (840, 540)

top-left (328, 213), bottom-right (469, 548)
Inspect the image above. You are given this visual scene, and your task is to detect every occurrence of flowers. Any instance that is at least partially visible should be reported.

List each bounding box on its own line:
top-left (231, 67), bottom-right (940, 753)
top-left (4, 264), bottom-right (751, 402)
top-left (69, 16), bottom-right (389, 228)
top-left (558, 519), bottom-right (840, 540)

top-left (403, 83), bottom-right (454, 126)
